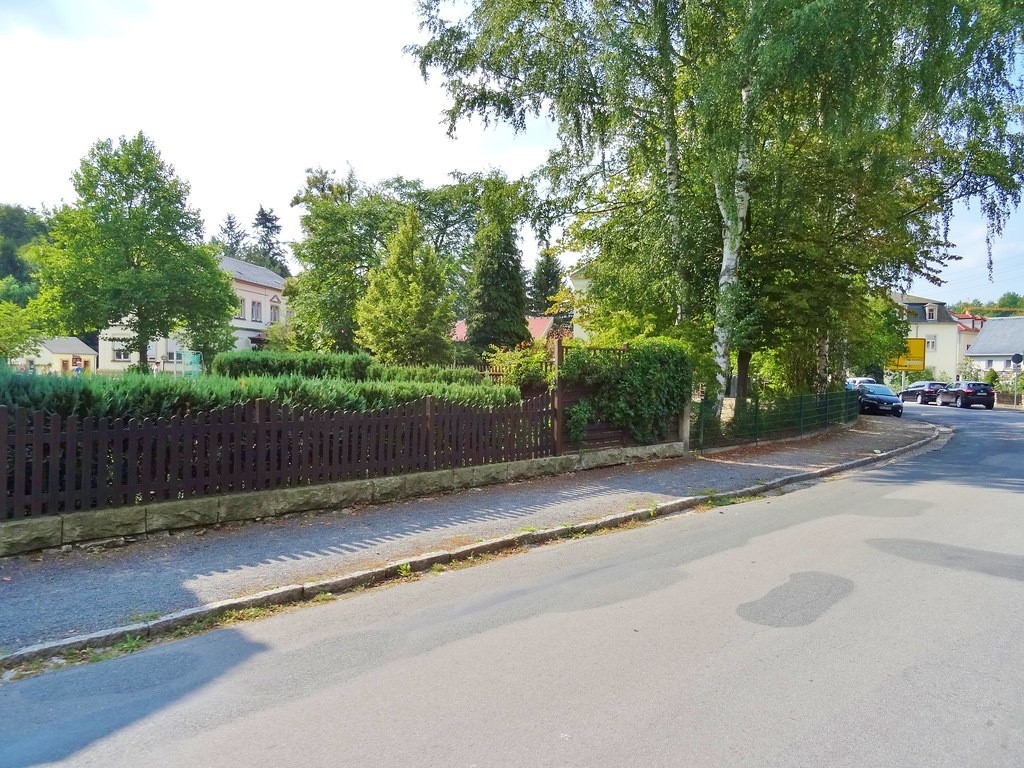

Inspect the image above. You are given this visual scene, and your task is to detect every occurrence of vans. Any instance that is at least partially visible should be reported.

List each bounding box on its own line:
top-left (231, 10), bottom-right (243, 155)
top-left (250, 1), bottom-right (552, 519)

top-left (849, 377), bottom-right (876, 388)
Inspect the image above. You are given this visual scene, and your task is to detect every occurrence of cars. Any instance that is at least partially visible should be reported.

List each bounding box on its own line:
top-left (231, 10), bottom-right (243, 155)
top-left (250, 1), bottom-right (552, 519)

top-left (936, 380), bottom-right (996, 410)
top-left (896, 381), bottom-right (951, 404)
top-left (854, 383), bottom-right (904, 417)
